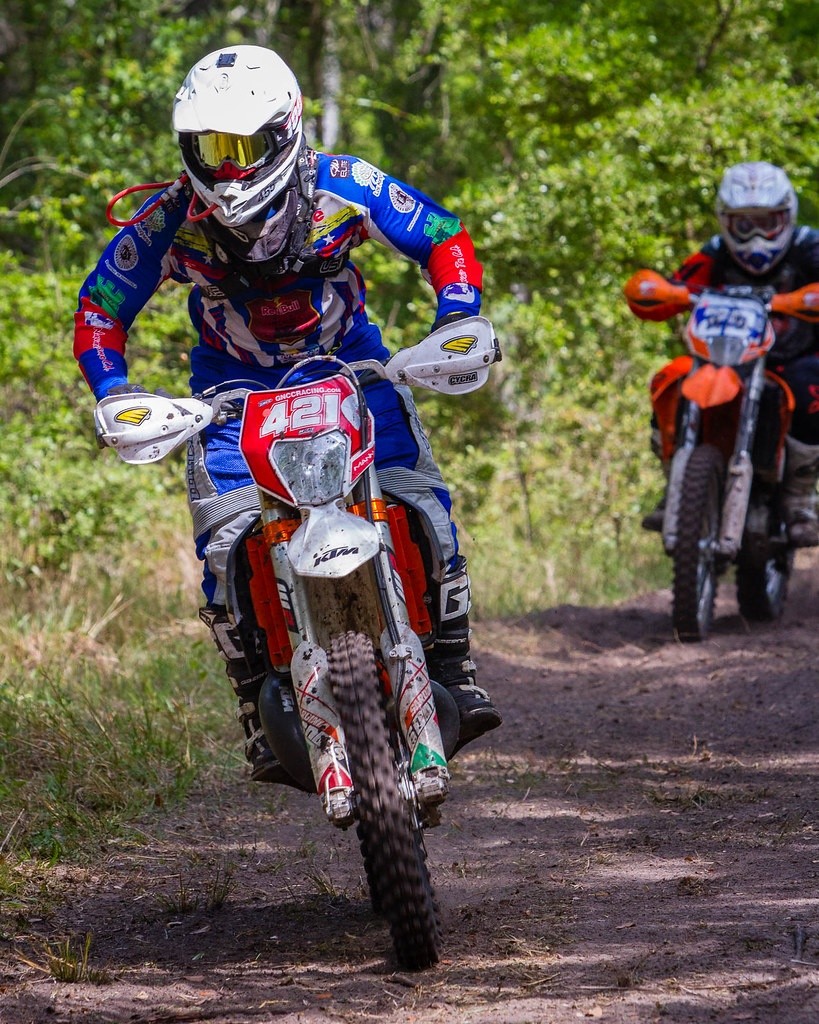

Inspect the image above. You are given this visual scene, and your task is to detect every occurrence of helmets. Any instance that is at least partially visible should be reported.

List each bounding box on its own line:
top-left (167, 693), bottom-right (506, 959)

top-left (172, 44), bottom-right (303, 227)
top-left (714, 162), bottom-right (798, 274)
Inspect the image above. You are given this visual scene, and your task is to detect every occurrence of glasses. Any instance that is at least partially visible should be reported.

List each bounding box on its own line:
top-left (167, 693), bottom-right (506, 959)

top-left (728, 212), bottom-right (785, 239)
top-left (191, 127), bottom-right (278, 171)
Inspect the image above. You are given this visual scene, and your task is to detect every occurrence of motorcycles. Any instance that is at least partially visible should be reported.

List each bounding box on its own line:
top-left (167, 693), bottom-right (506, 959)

top-left (93, 316), bottom-right (504, 972)
top-left (623, 269), bottom-right (819, 638)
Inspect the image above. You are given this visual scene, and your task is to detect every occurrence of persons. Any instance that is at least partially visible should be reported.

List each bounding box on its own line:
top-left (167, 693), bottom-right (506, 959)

top-left (75, 46), bottom-right (502, 779)
top-left (643, 161), bottom-right (819, 549)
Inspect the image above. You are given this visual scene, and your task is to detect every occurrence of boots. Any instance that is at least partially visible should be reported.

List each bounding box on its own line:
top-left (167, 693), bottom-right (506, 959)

top-left (196, 605), bottom-right (281, 783)
top-left (782, 435), bottom-right (819, 542)
top-left (426, 558), bottom-right (504, 731)
top-left (642, 487), bottom-right (664, 530)
